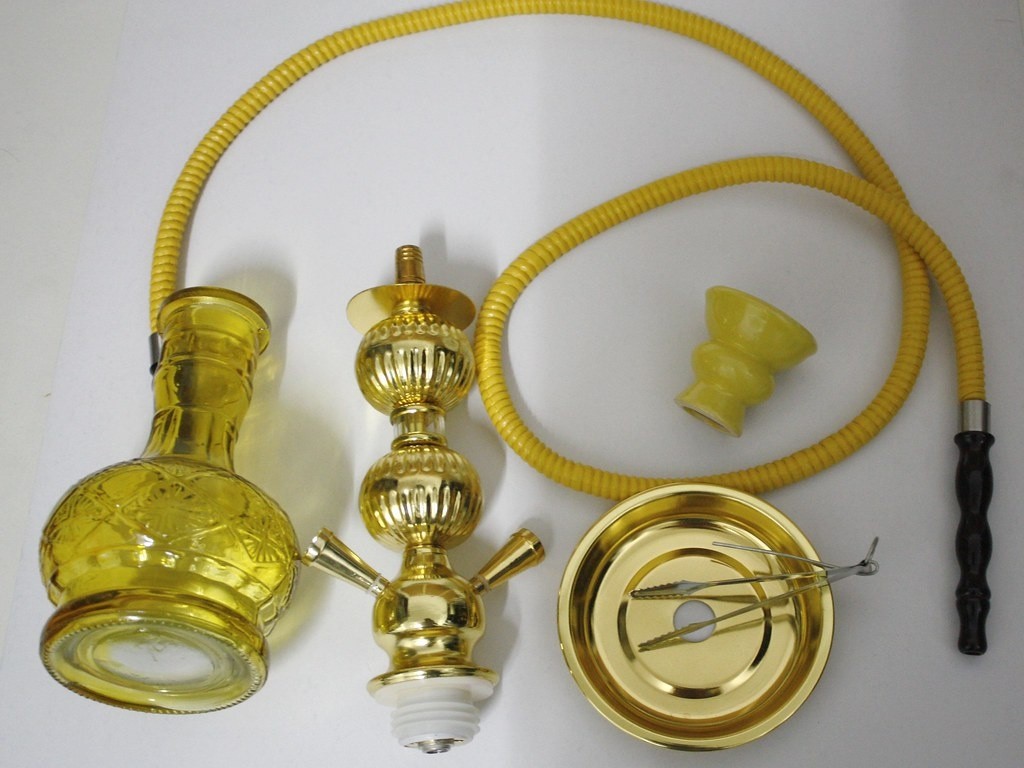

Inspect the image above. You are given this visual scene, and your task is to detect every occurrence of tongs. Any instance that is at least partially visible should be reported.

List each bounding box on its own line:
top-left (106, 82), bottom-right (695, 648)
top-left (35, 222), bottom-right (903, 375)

top-left (627, 536), bottom-right (881, 650)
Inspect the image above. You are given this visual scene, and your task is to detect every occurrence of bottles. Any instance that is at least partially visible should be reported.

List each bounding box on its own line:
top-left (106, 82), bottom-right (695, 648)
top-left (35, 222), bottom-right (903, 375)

top-left (672, 287), bottom-right (817, 441)
top-left (39, 287), bottom-right (301, 714)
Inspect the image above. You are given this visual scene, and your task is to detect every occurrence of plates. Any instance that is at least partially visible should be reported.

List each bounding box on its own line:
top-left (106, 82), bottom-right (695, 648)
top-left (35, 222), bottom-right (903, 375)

top-left (556, 482), bottom-right (836, 754)
